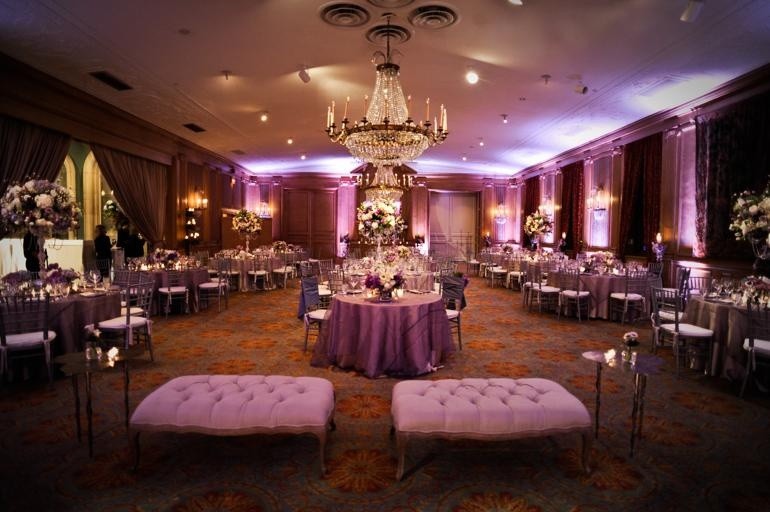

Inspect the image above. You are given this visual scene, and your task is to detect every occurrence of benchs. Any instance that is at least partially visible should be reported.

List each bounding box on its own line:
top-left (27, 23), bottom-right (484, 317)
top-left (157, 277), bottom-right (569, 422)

top-left (125, 374), bottom-right (338, 474)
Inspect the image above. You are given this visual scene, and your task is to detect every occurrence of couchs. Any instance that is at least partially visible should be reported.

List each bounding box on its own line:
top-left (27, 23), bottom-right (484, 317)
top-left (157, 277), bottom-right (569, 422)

top-left (390, 378), bottom-right (591, 480)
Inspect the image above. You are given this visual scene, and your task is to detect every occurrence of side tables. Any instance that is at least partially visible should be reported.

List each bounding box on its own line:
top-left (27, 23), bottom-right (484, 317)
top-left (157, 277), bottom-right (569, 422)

top-left (53, 345), bottom-right (144, 458)
top-left (581, 350), bottom-right (669, 456)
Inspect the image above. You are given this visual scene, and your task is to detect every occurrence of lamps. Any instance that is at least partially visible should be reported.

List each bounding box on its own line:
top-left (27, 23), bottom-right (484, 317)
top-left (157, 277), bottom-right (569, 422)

top-left (680, 1), bottom-right (708, 24)
top-left (317, 2), bottom-right (463, 162)
top-left (356, 165), bottom-right (414, 202)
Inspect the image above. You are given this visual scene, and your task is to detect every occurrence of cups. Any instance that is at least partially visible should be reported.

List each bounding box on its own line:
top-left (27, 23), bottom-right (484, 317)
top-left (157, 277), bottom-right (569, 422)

top-left (348, 275), bottom-right (359, 298)
top-left (712, 278), bottom-right (724, 298)
top-left (731, 293), bottom-right (742, 308)
top-left (90, 270), bottom-right (101, 288)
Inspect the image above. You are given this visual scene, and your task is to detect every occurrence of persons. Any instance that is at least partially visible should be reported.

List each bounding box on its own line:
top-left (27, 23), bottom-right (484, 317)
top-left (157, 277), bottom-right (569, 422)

top-left (23, 225), bottom-right (41, 279)
top-left (116, 220), bottom-right (130, 248)
top-left (94, 224), bottom-right (113, 281)
top-left (124, 228), bottom-right (146, 266)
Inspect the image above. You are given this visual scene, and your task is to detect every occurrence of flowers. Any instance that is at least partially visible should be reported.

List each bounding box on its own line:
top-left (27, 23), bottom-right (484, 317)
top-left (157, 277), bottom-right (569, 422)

top-left (231, 208), bottom-right (262, 236)
top-left (519, 209), bottom-right (554, 235)
top-left (153, 247), bottom-right (178, 262)
top-left (0, 174), bottom-right (82, 238)
top-left (104, 201), bottom-right (130, 230)
top-left (739, 274), bottom-right (770, 297)
top-left (272, 240), bottom-right (287, 250)
top-left (4, 271), bottom-right (31, 289)
top-left (38, 263), bottom-right (79, 291)
top-left (623, 332), bottom-right (641, 345)
top-left (384, 250), bottom-right (398, 266)
top-left (354, 201), bottom-right (397, 241)
top-left (80, 325), bottom-right (103, 342)
top-left (398, 246), bottom-right (410, 257)
top-left (726, 190), bottom-right (770, 261)
top-left (588, 253), bottom-right (607, 265)
top-left (503, 246), bottom-right (515, 253)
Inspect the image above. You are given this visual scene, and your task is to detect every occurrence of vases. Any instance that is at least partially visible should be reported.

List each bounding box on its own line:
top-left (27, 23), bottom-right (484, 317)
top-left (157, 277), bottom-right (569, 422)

top-left (375, 237), bottom-right (384, 264)
top-left (748, 255), bottom-right (770, 280)
top-left (85, 344), bottom-right (97, 360)
top-left (35, 240), bottom-right (51, 285)
top-left (625, 347), bottom-right (633, 361)
top-left (244, 234), bottom-right (252, 254)
top-left (378, 290), bottom-right (393, 302)
top-left (530, 234), bottom-right (541, 252)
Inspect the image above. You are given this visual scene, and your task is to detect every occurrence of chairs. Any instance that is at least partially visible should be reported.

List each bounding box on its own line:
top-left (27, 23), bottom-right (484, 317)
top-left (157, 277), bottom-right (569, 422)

top-left (298, 275), bottom-right (333, 354)
top-left (648, 262), bottom-right (663, 277)
top-left (651, 289), bottom-right (714, 377)
top-left (124, 272), bottom-right (154, 299)
top-left (248, 255), bottom-right (270, 291)
top-left (85, 282), bottom-right (154, 364)
top-left (558, 265), bottom-right (591, 325)
top-left (436, 276), bottom-right (469, 350)
top-left (160, 265), bottom-right (192, 320)
top-left (609, 266), bottom-right (647, 325)
top-left (272, 251), bottom-right (293, 288)
top-left (650, 266), bottom-right (690, 313)
top-left (210, 261), bottom-right (232, 309)
top-left (685, 277), bottom-right (708, 299)
top-left (478, 253), bottom-right (500, 277)
top-left (0, 295), bottom-right (57, 381)
top-left (528, 264), bottom-right (561, 316)
top-left (739, 298), bottom-right (770, 401)
top-left (197, 261), bottom-right (230, 313)
top-left (119, 273), bottom-right (156, 354)
top-left (506, 261), bottom-right (528, 292)
top-left (309, 259), bottom-right (329, 287)
top-left (519, 262), bottom-right (546, 309)
top-left (485, 254), bottom-right (507, 288)
top-left (227, 255), bottom-right (244, 290)
top-left (299, 262), bottom-right (331, 302)
top-left (643, 285), bottom-right (684, 352)
top-left (466, 249), bottom-right (480, 275)
